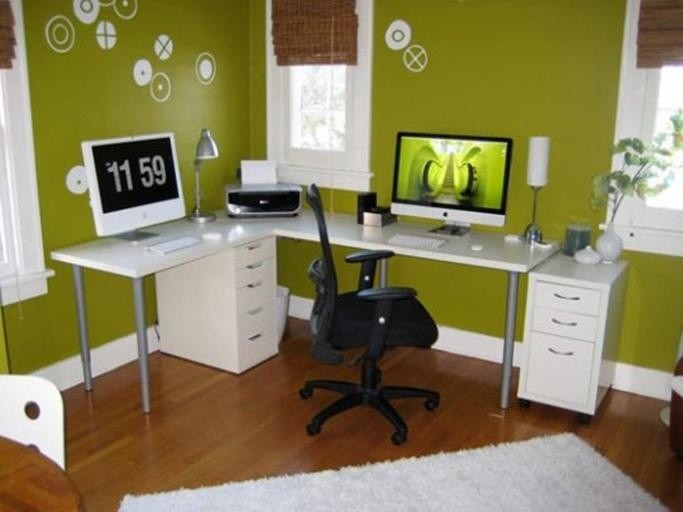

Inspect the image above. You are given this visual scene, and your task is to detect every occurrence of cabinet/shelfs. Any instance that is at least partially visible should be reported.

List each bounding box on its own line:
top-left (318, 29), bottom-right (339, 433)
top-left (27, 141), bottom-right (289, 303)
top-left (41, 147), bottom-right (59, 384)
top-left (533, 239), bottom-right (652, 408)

top-left (517, 247), bottom-right (627, 423)
top-left (154, 236), bottom-right (280, 374)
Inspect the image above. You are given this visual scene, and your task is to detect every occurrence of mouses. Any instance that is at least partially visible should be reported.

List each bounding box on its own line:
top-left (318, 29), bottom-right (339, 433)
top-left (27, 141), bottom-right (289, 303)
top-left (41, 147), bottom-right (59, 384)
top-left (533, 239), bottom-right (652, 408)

top-left (202, 231), bottom-right (221, 240)
top-left (470, 243), bottom-right (484, 252)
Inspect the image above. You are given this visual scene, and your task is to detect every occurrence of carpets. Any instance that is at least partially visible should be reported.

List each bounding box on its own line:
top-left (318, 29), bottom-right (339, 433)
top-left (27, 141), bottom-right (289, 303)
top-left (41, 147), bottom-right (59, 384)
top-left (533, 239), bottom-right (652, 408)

top-left (115, 432), bottom-right (676, 511)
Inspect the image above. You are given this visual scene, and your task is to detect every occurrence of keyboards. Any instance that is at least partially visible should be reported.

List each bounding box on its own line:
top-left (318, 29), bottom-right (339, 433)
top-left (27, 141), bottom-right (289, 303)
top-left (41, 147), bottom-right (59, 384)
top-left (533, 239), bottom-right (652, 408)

top-left (144, 235), bottom-right (202, 254)
top-left (388, 234), bottom-right (448, 251)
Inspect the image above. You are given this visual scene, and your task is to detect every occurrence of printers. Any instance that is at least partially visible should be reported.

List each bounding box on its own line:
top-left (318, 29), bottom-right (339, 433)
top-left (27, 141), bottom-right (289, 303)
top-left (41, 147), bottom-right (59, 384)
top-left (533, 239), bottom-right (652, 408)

top-left (223, 161), bottom-right (302, 218)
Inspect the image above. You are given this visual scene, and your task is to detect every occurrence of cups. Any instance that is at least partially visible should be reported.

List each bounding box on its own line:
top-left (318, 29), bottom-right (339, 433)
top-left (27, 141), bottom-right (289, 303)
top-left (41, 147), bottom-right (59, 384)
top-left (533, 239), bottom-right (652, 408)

top-left (564, 218), bottom-right (591, 257)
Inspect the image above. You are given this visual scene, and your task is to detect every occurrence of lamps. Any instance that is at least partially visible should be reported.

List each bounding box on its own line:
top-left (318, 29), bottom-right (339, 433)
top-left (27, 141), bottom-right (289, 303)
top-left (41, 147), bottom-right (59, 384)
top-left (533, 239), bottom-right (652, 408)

top-left (518, 136), bottom-right (550, 246)
top-left (188, 127), bottom-right (219, 225)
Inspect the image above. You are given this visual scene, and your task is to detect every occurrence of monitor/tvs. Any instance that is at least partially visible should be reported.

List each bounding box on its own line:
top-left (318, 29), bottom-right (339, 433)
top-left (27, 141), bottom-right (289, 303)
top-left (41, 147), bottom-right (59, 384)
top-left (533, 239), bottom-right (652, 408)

top-left (80, 132), bottom-right (186, 245)
top-left (391, 131), bottom-right (513, 238)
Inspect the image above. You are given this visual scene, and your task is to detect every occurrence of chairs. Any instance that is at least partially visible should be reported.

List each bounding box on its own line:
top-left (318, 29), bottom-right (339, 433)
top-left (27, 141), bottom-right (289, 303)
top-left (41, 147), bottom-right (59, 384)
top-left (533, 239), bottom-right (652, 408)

top-left (297, 182), bottom-right (439, 445)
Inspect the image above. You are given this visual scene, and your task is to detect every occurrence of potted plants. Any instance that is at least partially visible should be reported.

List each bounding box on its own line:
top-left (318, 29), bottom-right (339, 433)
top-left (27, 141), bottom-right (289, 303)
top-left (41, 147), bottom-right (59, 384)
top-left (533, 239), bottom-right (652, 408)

top-left (589, 96), bottom-right (682, 262)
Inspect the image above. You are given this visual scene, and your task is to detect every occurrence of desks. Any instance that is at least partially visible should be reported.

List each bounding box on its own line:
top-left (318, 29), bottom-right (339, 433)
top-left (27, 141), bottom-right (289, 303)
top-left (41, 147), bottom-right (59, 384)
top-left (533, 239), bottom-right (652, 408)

top-left (52, 206), bottom-right (559, 416)
